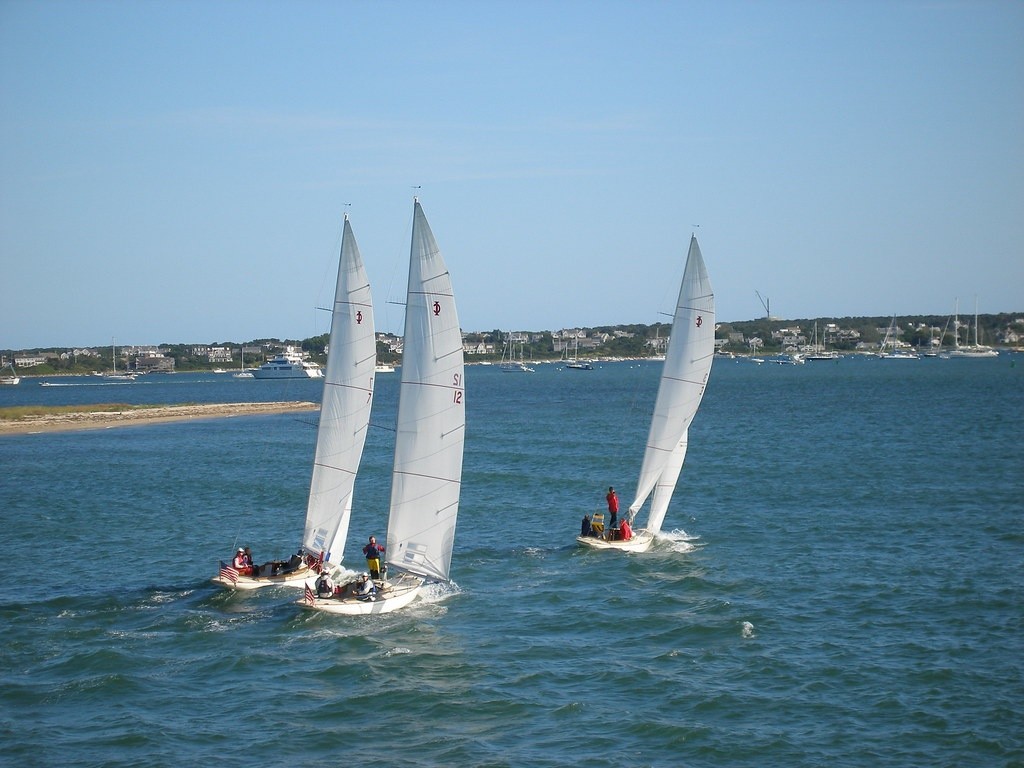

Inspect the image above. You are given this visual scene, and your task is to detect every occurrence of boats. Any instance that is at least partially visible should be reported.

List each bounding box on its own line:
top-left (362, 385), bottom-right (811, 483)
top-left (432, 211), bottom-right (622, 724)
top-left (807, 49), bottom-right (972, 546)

top-left (248, 344), bottom-right (326, 379)
top-left (0, 376), bottom-right (21, 385)
top-left (375, 360), bottom-right (396, 373)
top-left (214, 368), bottom-right (227, 374)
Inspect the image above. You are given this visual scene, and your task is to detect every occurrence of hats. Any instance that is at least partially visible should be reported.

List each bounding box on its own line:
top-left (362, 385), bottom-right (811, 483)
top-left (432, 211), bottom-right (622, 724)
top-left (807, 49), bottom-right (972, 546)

top-left (362, 572), bottom-right (368, 577)
top-left (323, 570), bottom-right (329, 574)
top-left (237, 547), bottom-right (244, 551)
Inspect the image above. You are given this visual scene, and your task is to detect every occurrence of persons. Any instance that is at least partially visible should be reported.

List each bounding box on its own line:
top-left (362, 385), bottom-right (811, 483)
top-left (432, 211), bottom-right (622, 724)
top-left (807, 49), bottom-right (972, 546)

top-left (234, 546), bottom-right (260, 577)
top-left (582, 514), bottom-right (598, 538)
top-left (314, 566), bottom-right (335, 598)
top-left (362, 536), bottom-right (384, 580)
top-left (354, 572), bottom-right (376, 603)
top-left (605, 487), bottom-right (619, 528)
top-left (619, 518), bottom-right (632, 540)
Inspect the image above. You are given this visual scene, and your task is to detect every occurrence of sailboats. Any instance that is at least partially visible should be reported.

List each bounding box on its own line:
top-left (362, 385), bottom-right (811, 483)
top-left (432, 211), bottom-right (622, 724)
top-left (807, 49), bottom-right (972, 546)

top-left (293, 184), bottom-right (467, 616)
top-left (231, 348), bottom-right (254, 379)
top-left (496, 290), bottom-right (999, 372)
top-left (210, 203), bottom-right (378, 592)
top-left (101, 336), bottom-right (135, 380)
top-left (572, 222), bottom-right (718, 554)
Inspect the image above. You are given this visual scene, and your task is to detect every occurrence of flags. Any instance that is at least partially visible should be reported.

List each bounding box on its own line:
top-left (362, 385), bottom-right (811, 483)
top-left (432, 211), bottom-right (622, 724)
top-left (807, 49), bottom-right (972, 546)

top-left (220, 560), bottom-right (239, 587)
top-left (305, 584), bottom-right (316, 606)
